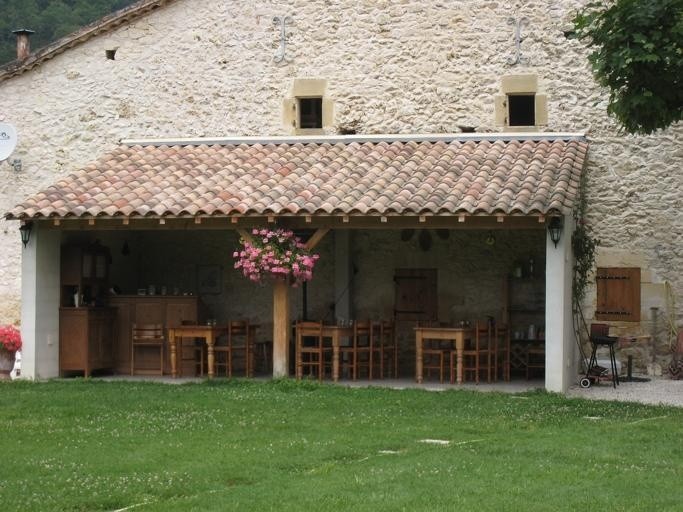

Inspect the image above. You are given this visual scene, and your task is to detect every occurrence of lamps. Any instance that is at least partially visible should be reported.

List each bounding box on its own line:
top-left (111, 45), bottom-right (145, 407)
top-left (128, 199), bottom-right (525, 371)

top-left (17, 221), bottom-right (31, 247)
top-left (547, 216), bottom-right (563, 249)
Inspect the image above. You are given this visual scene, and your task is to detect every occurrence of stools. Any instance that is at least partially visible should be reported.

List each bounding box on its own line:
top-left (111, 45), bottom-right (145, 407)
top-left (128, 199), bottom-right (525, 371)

top-left (524, 341), bottom-right (544, 382)
top-left (585, 332), bottom-right (618, 390)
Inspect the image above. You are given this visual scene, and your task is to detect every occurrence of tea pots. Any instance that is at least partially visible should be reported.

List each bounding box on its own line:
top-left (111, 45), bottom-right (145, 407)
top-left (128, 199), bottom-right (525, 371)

top-left (527, 324), bottom-right (536, 340)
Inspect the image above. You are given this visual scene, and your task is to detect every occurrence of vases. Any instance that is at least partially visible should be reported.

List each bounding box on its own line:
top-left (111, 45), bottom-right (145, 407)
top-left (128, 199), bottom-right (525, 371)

top-left (0, 347), bottom-right (15, 384)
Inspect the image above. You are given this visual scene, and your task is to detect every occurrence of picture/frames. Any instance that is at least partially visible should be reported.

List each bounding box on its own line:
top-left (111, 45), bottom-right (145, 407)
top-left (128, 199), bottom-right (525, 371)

top-left (195, 263), bottom-right (223, 296)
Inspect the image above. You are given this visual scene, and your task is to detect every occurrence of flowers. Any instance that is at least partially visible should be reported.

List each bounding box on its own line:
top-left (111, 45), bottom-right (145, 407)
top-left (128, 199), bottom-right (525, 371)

top-left (229, 225), bottom-right (317, 290)
top-left (1, 319), bottom-right (26, 357)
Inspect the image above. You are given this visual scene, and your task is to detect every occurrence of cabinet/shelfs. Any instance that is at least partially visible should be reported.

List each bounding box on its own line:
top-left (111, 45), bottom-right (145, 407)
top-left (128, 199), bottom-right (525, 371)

top-left (108, 293), bottom-right (201, 378)
top-left (500, 273), bottom-right (547, 383)
top-left (58, 240), bottom-right (120, 378)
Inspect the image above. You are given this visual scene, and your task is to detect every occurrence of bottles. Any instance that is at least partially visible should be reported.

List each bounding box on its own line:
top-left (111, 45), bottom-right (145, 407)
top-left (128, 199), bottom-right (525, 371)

top-left (339, 319), bottom-right (353, 327)
top-left (207, 318), bottom-right (216, 326)
top-left (460, 319), bottom-right (470, 329)
top-left (515, 330), bottom-right (526, 340)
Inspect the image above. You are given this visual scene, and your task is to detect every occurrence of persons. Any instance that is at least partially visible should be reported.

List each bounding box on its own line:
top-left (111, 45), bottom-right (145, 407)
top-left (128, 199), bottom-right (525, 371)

top-left (86, 282), bottom-right (104, 309)
